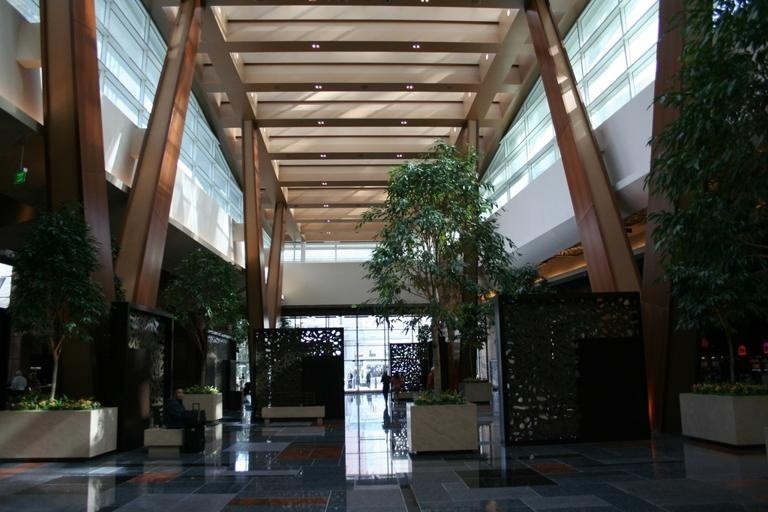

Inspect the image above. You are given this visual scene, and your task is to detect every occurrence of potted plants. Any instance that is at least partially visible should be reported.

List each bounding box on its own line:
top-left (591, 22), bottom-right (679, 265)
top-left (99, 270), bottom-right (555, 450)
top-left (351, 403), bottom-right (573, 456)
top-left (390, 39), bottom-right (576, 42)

top-left (0, 209), bottom-right (120, 460)
top-left (355, 140), bottom-right (557, 453)
top-left (643, 0), bottom-right (768, 447)
top-left (161, 248), bottom-right (250, 425)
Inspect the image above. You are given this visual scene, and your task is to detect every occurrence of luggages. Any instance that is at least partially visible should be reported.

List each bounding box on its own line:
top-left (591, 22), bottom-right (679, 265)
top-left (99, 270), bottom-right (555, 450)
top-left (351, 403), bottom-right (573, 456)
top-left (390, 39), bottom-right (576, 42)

top-left (181, 401), bottom-right (207, 453)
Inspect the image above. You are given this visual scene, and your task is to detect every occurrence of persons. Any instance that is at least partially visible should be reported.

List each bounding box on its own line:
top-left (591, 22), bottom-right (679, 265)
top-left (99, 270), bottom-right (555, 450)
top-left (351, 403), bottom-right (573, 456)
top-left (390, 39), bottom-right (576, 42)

top-left (426, 367), bottom-right (434, 390)
top-left (366, 372), bottom-right (370, 387)
top-left (381, 371), bottom-right (391, 402)
top-left (390, 373), bottom-right (401, 404)
top-left (167, 386), bottom-right (194, 455)
top-left (4, 367), bottom-right (41, 397)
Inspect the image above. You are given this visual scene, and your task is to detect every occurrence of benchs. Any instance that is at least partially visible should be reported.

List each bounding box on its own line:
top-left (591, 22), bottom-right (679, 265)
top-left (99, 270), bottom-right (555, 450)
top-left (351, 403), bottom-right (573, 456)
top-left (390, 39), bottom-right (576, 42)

top-left (144, 404), bottom-right (190, 456)
top-left (262, 406), bottom-right (325, 424)
